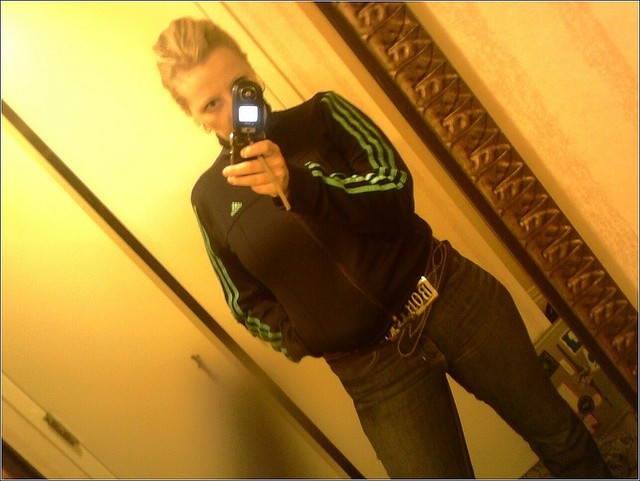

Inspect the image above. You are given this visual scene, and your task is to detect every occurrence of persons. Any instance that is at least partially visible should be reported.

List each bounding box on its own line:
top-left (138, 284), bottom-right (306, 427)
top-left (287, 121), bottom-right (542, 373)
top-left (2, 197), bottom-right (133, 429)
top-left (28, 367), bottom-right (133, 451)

top-left (151, 16), bottom-right (615, 481)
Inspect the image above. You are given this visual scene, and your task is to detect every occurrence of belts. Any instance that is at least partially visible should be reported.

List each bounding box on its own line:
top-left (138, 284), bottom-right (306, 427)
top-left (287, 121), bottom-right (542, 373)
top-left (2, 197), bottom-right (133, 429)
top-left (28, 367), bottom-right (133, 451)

top-left (371, 238), bottom-right (454, 359)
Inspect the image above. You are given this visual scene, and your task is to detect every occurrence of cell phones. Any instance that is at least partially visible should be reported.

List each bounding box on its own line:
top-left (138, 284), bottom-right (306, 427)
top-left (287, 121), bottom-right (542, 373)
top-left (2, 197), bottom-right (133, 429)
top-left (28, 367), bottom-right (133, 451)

top-left (230, 80), bottom-right (263, 165)
